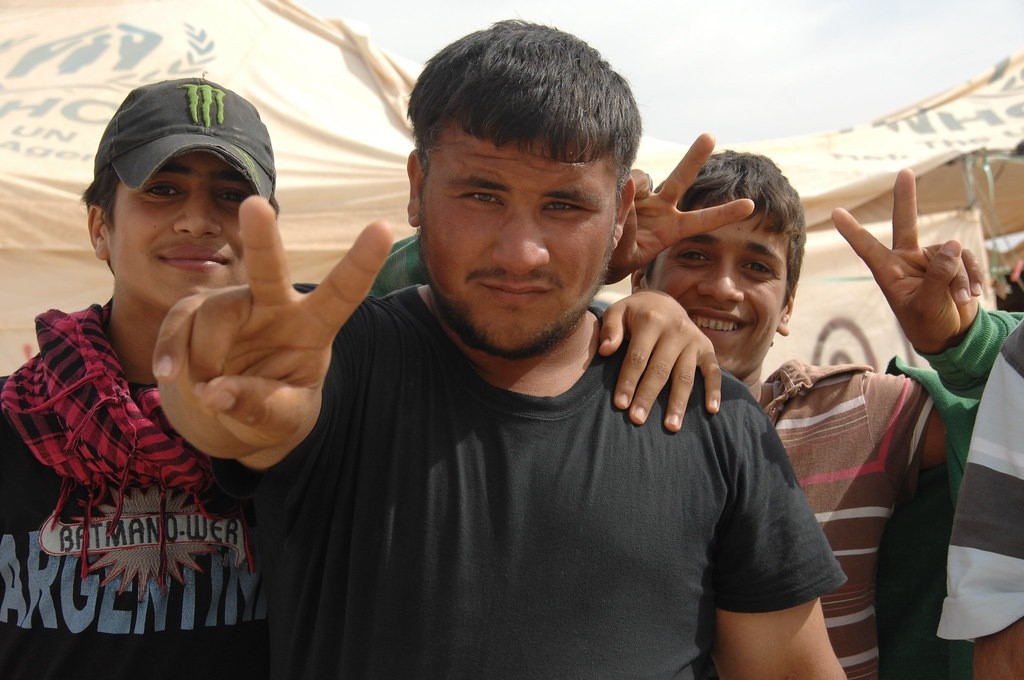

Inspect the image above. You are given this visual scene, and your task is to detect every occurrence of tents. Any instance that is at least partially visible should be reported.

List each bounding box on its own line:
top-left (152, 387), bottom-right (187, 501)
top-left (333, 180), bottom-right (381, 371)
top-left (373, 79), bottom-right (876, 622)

top-left (762, 53), bottom-right (1024, 376)
top-left (0, 0), bottom-right (413, 380)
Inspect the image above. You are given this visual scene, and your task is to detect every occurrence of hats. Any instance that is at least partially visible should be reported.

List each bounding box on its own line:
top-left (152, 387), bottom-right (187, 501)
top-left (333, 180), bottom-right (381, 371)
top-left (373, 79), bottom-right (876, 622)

top-left (94, 79), bottom-right (275, 205)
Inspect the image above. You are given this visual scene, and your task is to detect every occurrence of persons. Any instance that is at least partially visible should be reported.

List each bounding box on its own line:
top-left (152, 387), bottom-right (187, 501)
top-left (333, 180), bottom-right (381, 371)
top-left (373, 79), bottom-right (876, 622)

top-left (0, 78), bottom-right (722, 680)
top-left (150, 21), bottom-right (852, 680)
top-left (369, 134), bottom-right (1024, 680)
top-left (993, 262), bottom-right (1024, 312)
top-left (937, 316), bottom-right (1024, 680)
